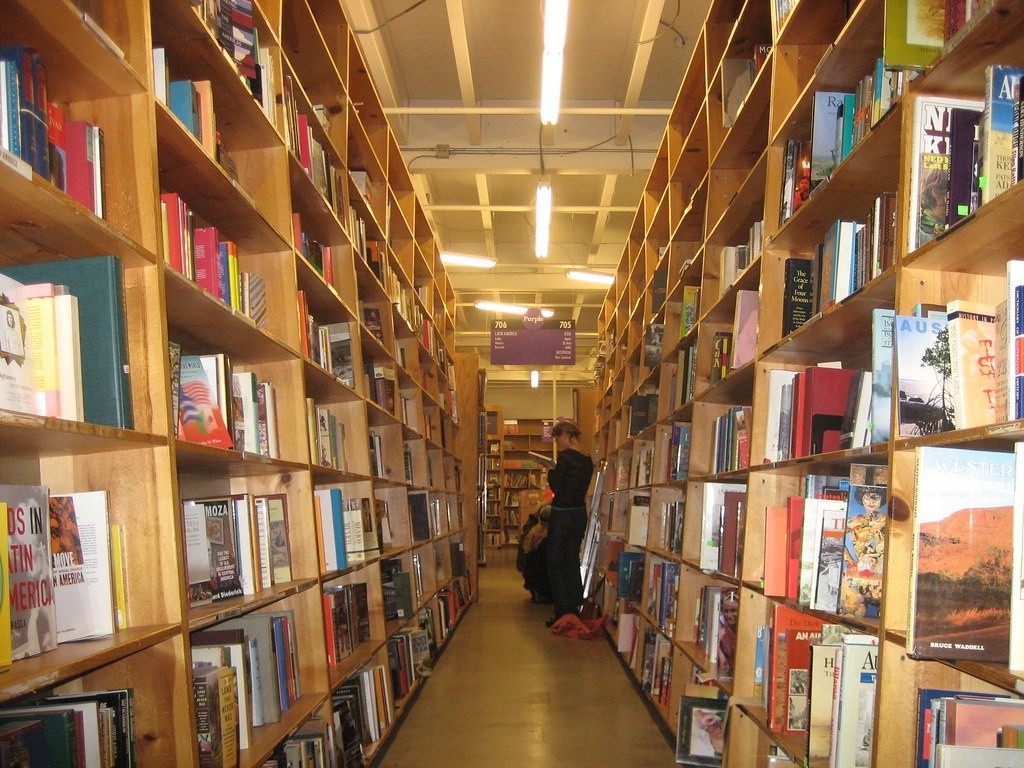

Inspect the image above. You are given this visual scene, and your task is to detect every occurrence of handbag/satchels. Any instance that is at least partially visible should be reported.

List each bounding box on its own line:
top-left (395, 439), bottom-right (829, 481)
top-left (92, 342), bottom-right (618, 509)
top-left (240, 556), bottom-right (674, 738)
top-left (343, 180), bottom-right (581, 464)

top-left (551, 613), bottom-right (611, 640)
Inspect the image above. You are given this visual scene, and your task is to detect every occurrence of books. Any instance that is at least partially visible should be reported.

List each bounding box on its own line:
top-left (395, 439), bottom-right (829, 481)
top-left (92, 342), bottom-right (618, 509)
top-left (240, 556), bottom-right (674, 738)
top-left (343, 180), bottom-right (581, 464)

top-left (0, 611), bottom-right (333, 768)
top-left (883, 0), bottom-right (1024, 768)
top-left (0, 483), bottom-right (129, 667)
top-left (297, 290), bottom-right (355, 392)
top-left (314, 489), bottom-right (375, 573)
top-left (589, 0), bottom-right (895, 768)
top-left (308, 398), bottom-right (350, 472)
top-left (153, 47), bottom-right (240, 184)
top-left (190, 0), bottom-right (278, 126)
top-left (283, 74), bottom-right (346, 229)
top-left (348, 171), bottom-right (472, 701)
top-left (322, 582), bottom-right (395, 768)
top-left (0, 44), bottom-right (106, 221)
top-left (0, 253), bottom-right (136, 431)
top-left (168, 341), bottom-right (280, 459)
top-left (161, 192), bottom-right (266, 329)
top-left (181, 493), bottom-right (292, 610)
top-left (292, 211), bottom-right (333, 285)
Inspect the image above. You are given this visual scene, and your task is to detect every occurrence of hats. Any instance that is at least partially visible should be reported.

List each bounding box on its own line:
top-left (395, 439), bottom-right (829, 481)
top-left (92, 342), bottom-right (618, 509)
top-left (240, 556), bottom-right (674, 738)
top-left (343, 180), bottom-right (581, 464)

top-left (719, 587), bottom-right (739, 602)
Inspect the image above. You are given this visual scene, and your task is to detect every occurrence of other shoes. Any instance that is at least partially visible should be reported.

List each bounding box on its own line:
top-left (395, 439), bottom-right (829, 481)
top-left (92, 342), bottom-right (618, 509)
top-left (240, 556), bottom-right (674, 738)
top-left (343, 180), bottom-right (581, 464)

top-left (545, 616), bottom-right (558, 627)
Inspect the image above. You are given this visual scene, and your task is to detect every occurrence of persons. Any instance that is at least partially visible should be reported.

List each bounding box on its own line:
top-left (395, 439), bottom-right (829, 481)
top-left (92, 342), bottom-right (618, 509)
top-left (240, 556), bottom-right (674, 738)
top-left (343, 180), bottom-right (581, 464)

top-left (545, 420), bottom-right (593, 628)
top-left (515, 501), bottom-right (565, 604)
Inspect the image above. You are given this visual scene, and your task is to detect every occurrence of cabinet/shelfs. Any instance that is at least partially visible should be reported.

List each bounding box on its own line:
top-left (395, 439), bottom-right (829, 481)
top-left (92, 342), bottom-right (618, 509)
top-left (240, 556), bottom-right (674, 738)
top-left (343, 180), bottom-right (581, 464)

top-left (0, 0), bottom-right (456, 768)
top-left (579, 0), bottom-right (1024, 768)
top-left (457, 353), bottom-right (595, 603)
